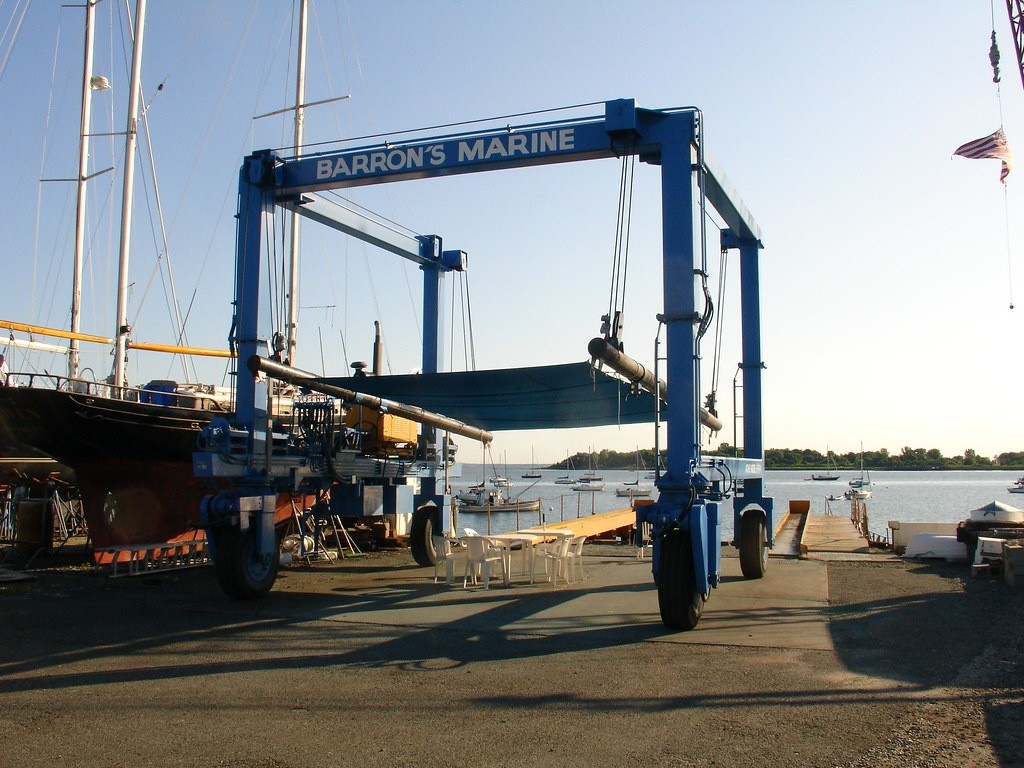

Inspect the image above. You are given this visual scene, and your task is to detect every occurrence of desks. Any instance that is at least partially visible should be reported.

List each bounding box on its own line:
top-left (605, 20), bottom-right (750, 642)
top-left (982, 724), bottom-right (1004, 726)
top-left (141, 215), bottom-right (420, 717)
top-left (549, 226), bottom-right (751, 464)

top-left (516, 529), bottom-right (574, 578)
top-left (480, 535), bottom-right (539, 584)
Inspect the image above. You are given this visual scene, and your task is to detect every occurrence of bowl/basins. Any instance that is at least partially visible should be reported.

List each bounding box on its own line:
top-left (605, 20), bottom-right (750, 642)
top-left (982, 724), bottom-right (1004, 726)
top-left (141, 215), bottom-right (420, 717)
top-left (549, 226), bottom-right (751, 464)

top-left (179, 395), bottom-right (196, 408)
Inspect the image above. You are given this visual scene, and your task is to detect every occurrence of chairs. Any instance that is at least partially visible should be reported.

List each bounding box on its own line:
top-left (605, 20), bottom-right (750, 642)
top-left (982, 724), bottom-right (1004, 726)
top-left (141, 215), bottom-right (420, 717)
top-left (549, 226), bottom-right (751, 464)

top-left (463, 536), bottom-right (510, 590)
top-left (530, 534), bottom-right (575, 586)
top-left (549, 535), bottom-right (588, 584)
top-left (432, 535), bottom-right (470, 584)
top-left (464, 527), bottom-right (501, 580)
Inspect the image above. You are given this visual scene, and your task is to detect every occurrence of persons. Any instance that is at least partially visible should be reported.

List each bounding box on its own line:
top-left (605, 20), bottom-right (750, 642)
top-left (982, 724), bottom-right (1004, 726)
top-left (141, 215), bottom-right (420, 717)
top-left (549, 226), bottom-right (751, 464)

top-left (830, 494), bottom-right (833, 500)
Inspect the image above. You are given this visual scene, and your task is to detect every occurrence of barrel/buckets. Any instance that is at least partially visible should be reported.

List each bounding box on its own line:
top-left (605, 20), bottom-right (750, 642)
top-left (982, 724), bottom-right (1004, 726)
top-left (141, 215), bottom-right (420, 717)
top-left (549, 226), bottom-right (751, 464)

top-left (128, 391), bottom-right (138, 402)
top-left (16, 499), bottom-right (54, 552)
top-left (150, 385), bottom-right (175, 406)
top-left (139, 390), bottom-right (149, 403)
top-left (73, 378), bottom-right (85, 392)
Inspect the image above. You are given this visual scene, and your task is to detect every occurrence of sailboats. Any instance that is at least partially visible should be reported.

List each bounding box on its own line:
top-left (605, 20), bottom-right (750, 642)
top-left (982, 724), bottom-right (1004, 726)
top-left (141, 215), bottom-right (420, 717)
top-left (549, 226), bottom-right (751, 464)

top-left (0, 0), bottom-right (347, 568)
top-left (844, 441), bottom-right (876, 500)
top-left (521, 446), bottom-right (543, 478)
top-left (489, 453), bottom-right (506, 483)
top-left (556, 443), bottom-right (605, 491)
top-left (614, 445), bottom-right (652, 497)
top-left (812, 445), bottom-right (840, 480)
top-left (494, 450), bottom-right (513, 487)
top-left (644, 449), bottom-right (667, 479)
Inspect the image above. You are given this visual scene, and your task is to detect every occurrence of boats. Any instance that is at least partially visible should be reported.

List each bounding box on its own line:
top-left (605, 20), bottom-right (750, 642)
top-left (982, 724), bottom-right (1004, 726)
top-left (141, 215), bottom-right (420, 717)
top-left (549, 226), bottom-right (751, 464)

top-left (622, 482), bottom-right (637, 485)
top-left (455, 485), bottom-right (540, 513)
top-left (828, 495), bottom-right (843, 500)
top-left (1007, 477), bottom-right (1024, 493)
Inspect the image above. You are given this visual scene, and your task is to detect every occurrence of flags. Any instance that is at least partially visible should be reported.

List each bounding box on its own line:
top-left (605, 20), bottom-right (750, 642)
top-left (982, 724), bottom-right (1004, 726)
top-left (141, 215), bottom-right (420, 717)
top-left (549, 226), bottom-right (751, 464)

top-left (954, 124), bottom-right (1012, 185)
